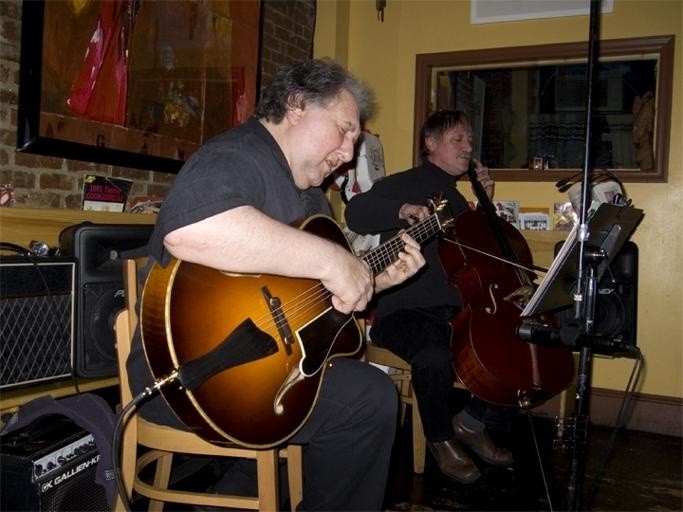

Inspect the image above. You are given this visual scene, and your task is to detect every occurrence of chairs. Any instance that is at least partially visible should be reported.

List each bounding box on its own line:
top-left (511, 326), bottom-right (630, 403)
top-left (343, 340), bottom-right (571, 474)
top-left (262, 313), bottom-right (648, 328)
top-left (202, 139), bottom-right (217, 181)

top-left (108, 254), bottom-right (304, 511)
top-left (356, 316), bottom-right (427, 475)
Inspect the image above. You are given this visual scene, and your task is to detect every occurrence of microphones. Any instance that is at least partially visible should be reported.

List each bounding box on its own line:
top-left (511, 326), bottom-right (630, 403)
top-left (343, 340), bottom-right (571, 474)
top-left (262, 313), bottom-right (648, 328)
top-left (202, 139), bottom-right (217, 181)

top-left (556, 165), bottom-right (626, 205)
top-left (515, 323), bottom-right (640, 359)
top-left (559, 173), bottom-right (609, 193)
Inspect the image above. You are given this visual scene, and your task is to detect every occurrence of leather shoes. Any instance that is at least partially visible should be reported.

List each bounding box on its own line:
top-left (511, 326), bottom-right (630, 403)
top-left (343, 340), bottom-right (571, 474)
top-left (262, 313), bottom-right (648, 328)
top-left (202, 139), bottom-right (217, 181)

top-left (426, 415), bottom-right (513, 484)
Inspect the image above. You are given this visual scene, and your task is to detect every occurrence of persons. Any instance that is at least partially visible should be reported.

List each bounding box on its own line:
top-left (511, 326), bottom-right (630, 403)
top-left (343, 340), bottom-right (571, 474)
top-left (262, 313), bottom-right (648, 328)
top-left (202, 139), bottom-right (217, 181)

top-left (343, 105), bottom-right (514, 485)
top-left (125, 55), bottom-right (427, 512)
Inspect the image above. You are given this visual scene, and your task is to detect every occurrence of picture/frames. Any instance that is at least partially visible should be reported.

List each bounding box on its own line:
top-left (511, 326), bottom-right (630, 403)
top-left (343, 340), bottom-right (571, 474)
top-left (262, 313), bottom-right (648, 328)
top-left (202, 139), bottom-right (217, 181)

top-left (16, 0), bottom-right (265, 174)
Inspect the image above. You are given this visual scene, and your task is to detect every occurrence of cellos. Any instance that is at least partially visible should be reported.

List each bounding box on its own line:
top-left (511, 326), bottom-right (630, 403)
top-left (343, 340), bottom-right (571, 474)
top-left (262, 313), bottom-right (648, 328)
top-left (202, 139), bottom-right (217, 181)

top-left (436, 158), bottom-right (574, 410)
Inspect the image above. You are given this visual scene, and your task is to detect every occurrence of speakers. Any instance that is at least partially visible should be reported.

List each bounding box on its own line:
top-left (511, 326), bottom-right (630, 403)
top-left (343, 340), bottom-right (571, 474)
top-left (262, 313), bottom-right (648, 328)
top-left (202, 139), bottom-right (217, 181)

top-left (0, 412), bottom-right (100, 512)
top-left (0, 255), bottom-right (77, 391)
top-left (58, 221), bottom-right (155, 380)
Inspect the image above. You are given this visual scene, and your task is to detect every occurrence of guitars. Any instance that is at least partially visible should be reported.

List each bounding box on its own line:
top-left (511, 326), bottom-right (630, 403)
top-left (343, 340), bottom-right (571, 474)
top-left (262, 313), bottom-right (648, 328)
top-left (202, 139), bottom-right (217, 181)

top-left (137, 194), bottom-right (459, 450)
